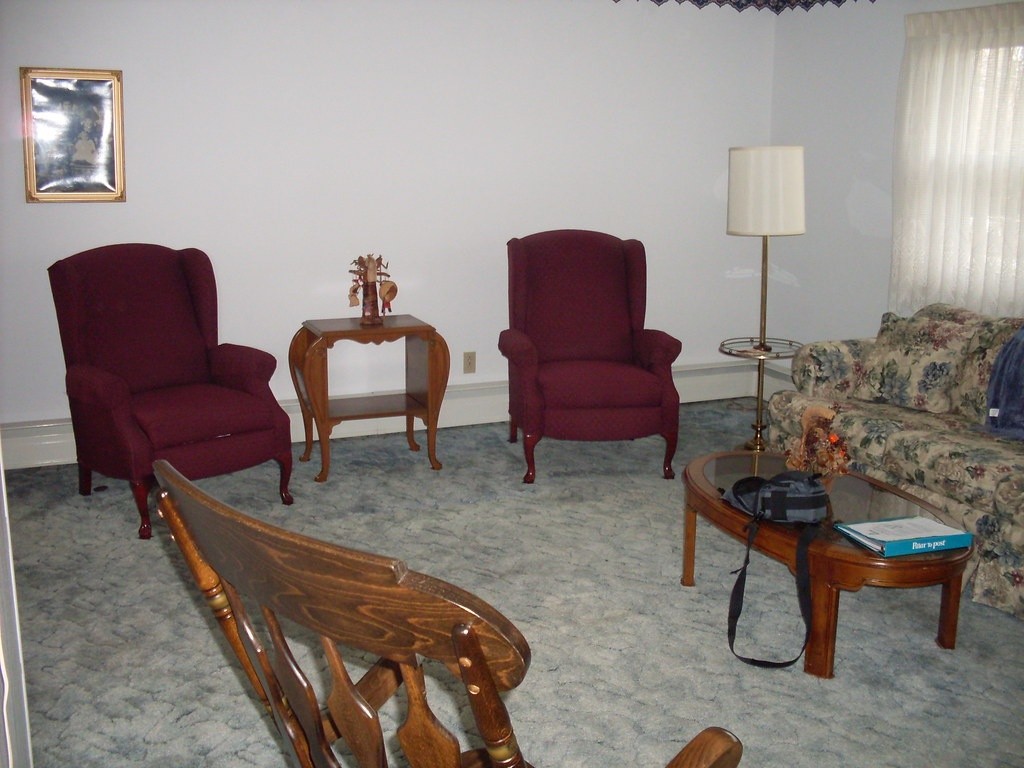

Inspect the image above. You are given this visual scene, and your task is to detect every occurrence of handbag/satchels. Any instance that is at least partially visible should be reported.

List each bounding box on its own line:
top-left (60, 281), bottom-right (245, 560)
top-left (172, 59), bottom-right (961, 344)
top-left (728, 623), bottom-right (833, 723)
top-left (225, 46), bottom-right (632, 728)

top-left (718, 469), bottom-right (830, 525)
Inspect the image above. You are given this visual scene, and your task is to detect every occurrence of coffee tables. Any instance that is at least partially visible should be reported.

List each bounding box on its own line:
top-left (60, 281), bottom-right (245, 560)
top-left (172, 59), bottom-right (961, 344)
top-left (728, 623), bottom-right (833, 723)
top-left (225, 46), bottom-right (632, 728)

top-left (680, 451), bottom-right (975, 679)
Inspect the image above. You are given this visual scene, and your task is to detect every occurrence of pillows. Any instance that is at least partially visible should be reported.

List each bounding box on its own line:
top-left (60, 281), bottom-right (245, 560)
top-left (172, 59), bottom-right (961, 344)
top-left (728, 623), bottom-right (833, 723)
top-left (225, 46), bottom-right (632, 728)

top-left (851, 311), bottom-right (976, 413)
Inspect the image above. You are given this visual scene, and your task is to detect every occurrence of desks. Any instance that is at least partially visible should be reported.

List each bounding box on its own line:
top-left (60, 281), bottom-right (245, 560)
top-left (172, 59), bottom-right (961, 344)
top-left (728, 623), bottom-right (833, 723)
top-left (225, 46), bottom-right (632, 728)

top-left (288, 314), bottom-right (451, 483)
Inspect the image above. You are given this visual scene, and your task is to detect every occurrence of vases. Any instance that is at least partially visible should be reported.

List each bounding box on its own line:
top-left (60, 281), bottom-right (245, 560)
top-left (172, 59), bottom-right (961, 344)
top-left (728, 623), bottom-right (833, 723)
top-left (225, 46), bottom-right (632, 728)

top-left (812, 472), bottom-right (833, 494)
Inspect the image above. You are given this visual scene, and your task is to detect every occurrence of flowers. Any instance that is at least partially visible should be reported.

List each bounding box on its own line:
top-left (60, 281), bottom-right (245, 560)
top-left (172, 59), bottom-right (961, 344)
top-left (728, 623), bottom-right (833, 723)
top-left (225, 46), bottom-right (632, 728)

top-left (785, 402), bottom-right (849, 478)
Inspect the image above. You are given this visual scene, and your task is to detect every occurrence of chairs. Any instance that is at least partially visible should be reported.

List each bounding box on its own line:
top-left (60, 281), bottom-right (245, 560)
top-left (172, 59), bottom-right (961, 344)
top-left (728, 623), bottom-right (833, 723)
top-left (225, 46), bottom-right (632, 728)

top-left (497, 229), bottom-right (681, 483)
top-left (150, 459), bottom-right (742, 768)
top-left (47, 243), bottom-right (294, 540)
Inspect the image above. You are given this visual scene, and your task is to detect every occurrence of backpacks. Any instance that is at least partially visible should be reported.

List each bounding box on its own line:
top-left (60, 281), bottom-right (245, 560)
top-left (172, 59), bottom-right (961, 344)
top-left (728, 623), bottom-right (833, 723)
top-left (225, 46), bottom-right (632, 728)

top-left (978, 327), bottom-right (1024, 440)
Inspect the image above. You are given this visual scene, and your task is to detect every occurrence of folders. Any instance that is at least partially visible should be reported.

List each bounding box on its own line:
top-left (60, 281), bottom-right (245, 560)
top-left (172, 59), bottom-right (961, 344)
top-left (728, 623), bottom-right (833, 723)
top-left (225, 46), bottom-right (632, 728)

top-left (834, 514), bottom-right (972, 557)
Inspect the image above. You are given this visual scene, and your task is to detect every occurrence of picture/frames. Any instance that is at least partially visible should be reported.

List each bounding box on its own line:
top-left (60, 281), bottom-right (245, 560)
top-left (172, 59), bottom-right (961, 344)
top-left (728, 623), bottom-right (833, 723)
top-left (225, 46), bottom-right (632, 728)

top-left (19, 67), bottom-right (125, 204)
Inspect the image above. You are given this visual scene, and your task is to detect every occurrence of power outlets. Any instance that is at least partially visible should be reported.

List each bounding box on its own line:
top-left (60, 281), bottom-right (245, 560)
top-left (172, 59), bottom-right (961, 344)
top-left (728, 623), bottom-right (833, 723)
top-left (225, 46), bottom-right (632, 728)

top-left (463, 352), bottom-right (476, 374)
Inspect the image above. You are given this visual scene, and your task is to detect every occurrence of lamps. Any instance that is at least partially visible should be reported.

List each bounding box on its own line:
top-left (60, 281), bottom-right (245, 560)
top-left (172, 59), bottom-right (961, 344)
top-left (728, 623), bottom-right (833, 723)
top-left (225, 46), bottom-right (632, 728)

top-left (718, 145), bottom-right (805, 451)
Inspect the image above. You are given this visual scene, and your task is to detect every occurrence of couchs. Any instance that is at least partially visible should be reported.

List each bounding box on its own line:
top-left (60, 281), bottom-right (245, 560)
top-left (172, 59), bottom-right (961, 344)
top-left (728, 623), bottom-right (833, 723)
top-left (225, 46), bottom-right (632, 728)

top-left (757, 302), bottom-right (1024, 623)
